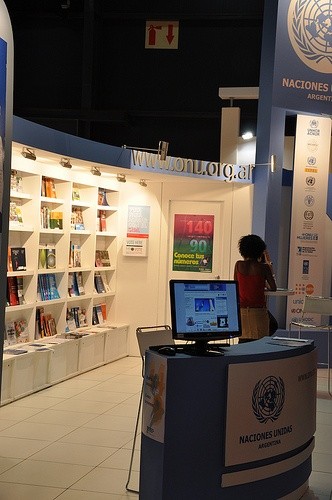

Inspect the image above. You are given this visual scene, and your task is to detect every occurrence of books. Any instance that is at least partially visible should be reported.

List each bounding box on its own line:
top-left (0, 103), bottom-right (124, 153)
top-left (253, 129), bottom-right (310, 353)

top-left (3, 165), bottom-right (120, 359)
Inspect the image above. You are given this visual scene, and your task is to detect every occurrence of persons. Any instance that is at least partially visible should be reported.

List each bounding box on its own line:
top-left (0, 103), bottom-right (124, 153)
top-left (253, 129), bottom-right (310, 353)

top-left (233, 234), bottom-right (277, 344)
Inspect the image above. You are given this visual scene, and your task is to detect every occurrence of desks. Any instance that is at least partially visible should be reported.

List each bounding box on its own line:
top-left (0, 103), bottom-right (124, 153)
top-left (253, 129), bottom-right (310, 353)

top-left (263, 287), bottom-right (295, 309)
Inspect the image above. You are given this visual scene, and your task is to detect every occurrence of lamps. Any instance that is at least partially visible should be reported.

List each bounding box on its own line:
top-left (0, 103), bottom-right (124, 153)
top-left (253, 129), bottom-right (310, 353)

top-left (60, 157), bottom-right (72, 169)
top-left (115, 174), bottom-right (126, 183)
top-left (139, 179), bottom-right (147, 187)
top-left (248, 154), bottom-right (275, 179)
top-left (21, 147), bottom-right (37, 160)
top-left (90, 166), bottom-right (101, 176)
top-left (122, 141), bottom-right (169, 161)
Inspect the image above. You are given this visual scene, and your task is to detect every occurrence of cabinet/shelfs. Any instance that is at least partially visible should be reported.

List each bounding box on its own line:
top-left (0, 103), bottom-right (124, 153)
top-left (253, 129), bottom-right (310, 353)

top-left (0, 157), bottom-right (129, 407)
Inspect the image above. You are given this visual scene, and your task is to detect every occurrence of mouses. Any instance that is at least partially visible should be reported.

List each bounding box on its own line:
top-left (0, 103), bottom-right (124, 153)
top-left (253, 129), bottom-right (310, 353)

top-left (158, 346), bottom-right (176, 356)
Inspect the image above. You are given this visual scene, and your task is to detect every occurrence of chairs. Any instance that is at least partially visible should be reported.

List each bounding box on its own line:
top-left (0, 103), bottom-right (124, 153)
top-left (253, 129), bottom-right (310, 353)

top-left (289, 295), bottom-right (332, 397)
top-left (125, 324), bottom-right (176, 494)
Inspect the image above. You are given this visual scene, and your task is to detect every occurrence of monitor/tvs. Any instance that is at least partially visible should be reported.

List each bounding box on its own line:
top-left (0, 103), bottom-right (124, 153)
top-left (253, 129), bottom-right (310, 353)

top-left (169, 279), bottom-right (242, 356)
